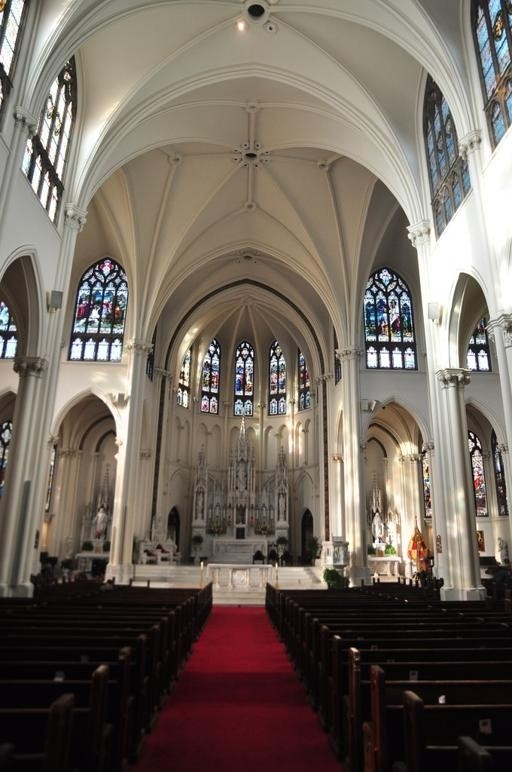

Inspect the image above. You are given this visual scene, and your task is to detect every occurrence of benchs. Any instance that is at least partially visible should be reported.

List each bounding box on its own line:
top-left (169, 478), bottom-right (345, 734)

top-left (2, 573), bottom-right (220, 771)
top-left (260, 564), bottom-right (512, 772)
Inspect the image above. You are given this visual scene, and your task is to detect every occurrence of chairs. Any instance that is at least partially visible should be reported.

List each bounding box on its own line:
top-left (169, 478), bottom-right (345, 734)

top-left (252, 541), bottom-right (265, 566)
top-left (162, 539), bottom-right (185, 565)
top-left (135, 540), bottom-right (171, 565)
top-left (281, 548), bottom-right (293, 569)
top-left (268, 541), bottom-right (279, 564)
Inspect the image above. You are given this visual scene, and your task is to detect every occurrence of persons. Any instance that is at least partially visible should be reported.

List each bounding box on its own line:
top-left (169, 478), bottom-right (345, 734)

top-left (371, 509), bottom-right (384, 545)
top-left (92, 504), bottom-right (108, 540)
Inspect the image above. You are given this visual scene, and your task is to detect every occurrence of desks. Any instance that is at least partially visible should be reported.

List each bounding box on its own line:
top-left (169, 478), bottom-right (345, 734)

top-left (74, 551), bottom-right (110, 574)
top-left (364, 556), bottom-right (401, 580)
